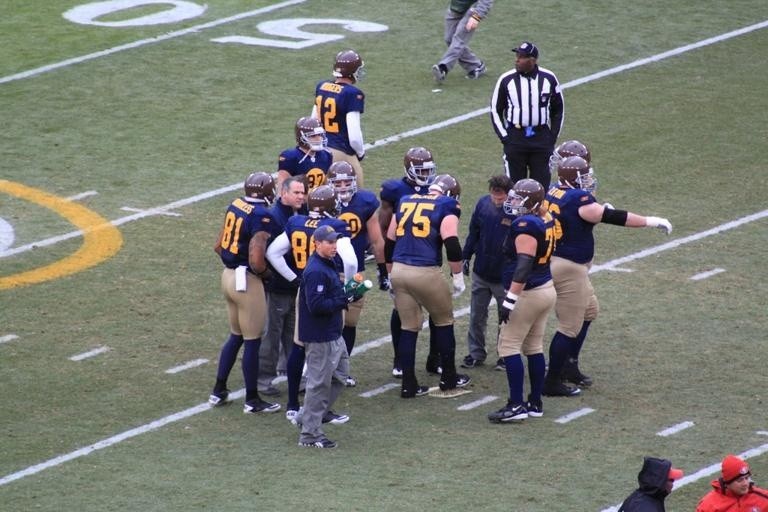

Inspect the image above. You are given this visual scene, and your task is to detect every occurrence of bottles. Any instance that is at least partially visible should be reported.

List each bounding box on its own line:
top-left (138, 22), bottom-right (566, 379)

top-left (345, 273), bottom-right (362, 292)
top-left (357, 280), bottom-right (373, 295)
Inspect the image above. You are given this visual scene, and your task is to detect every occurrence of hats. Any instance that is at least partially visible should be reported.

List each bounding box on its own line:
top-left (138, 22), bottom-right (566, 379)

top-left (314, 225), bottom-right (344, 242)
top-left (667, 466), bottom-right (684, 480)
top-left (511, 42), bottom-right (539, 59)
top-left (721, 455), bottom-right (752, 484)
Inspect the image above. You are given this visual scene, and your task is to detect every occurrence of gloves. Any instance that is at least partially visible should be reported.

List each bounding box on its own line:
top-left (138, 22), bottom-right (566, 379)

top-left (498, 297), bottom-right (516, 325)
top-left (344, 293), bottom-right (363, 304)
top-left (462, 258), bottom-right (470, 277)
top-left (376, 262), bottom-right (390, 290)
top-left (384, 273), bottom-right (396, 302)
top-left (645, 216), bottom-right (674, 236)
top-left (449, 270), bottom-right (466, 300)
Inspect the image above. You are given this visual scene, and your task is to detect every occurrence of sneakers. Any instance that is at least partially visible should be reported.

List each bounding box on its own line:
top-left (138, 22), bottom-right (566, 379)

top-left (439, 373), bottom-right (471, 390)
top-left (561, 373), bottom-right (593, 386)
top-left (494, 358), bottom-right (507, 371)
top-left (260, 387), bottom-right (281, 395)
top-left (488, 397), bottom-right (529, 423)
top-left (208, 389), bottom-right (234, 405)
top-left (431, 65), bottom-right (446, 84)
top-left (426, 366), bottom-right (443, 374)
top-left (243, 397), bottom-right (281, 414)
top-left (461, 355), bottom-right (485, 368)
top-left (322, 412), bottom-right (350, 425)
top-left (401, 385), bottom-right (430, 398)
top-left (525, 399), bottom-right (544, 417)
top-left (298, 438), bottom-right (337, 449)
top-left (464, 61), bottom-right (486, 81)
top-left (392, 367), bottom-right (404, 377)
top-left (286, 406), bottom-right (303, 420)
top-left (346, 377), bottom-right (355, 386)
top-left (542, 383), bottom-right (581, 396)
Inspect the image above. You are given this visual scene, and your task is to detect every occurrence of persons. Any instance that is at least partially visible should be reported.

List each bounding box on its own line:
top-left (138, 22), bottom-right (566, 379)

top-left (464, 175), bottom-right (558, 422)
top-left (311, 46), bottom-right (369, 193)
top-left (696, 456), bottom-right (768, 511)
top-left (205, 115), bottom-right (389, 450)
top-left (488, 40), bottom-right (567, 198)
top-left (430, 0), bottom-right (491, 85)
top-left (544, 140), bottom-right (672, 399)
top-left (618, 458), bottom-right (684, 510)
top-left (377, 144), bottom-right (471, 401)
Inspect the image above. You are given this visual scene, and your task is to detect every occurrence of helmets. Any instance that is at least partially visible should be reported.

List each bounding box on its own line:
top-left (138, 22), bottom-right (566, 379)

top-left (244, 171), bottom-right (277, 204)
top-left (327, 161), bottom-right (357, 204)
top-left (403, 147), bottom-right (437, 187)
top-left (503, 178), bottom-right (546, 217)
top-left (294, 117), bottom-right (329, 152)
top-left (428, 174), bottom-right (462, 202)
top-left (557, 155), bottom-right (598, 193)
top-left (332, 49), bottom-right (365, 85)
top-left (308, 183), bottom-right (342, 217)
top-left (548, 140), bottom-right (591, 173)
